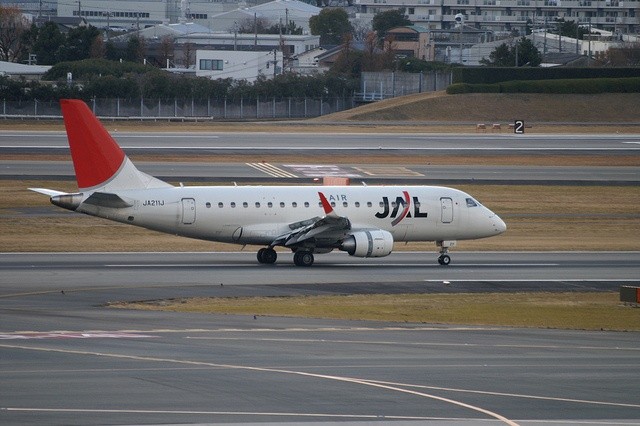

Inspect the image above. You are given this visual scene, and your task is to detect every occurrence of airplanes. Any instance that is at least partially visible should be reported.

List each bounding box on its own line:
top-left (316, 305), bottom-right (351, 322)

top-left (22, 99), bottom-right (507, 265)
